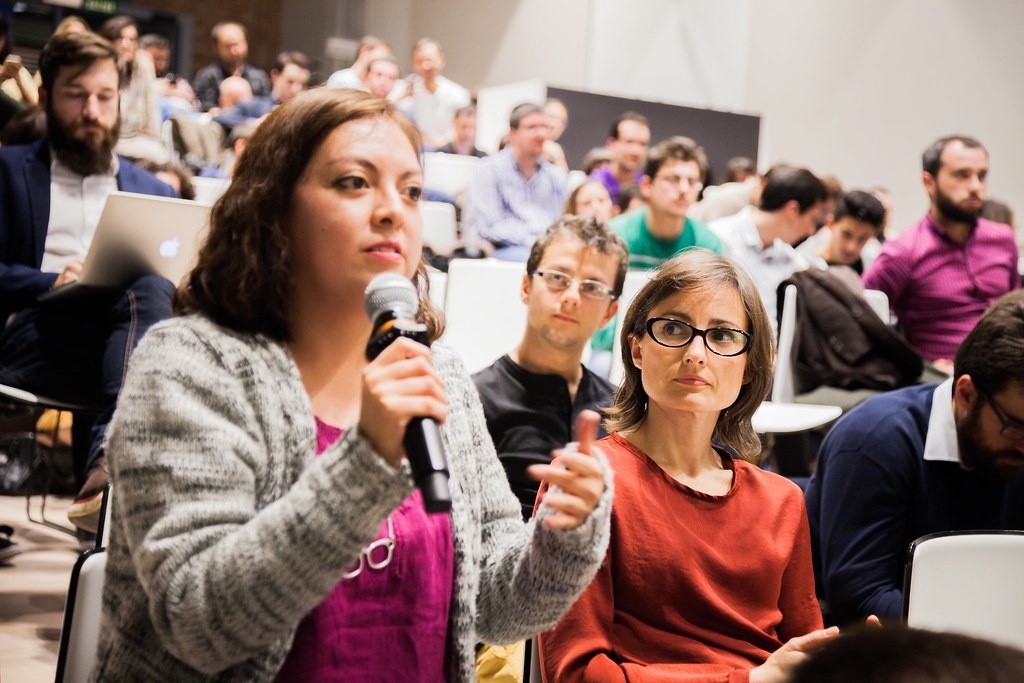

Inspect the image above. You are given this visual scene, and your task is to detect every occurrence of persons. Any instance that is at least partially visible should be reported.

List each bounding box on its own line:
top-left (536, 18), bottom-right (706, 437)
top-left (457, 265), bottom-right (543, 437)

top-left (92, 86), bottom-right (615, 683)
top-left (0, 8), bottom-right (1024, 682)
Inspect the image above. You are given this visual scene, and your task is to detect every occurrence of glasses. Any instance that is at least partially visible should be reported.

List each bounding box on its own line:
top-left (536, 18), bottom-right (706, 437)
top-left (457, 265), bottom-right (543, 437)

top-left (974, 381), bottom-right (1024, 441)
top-left (638, 318), bottom-right (753, 357)
top-left (531, 270), bottom-right (615, 300)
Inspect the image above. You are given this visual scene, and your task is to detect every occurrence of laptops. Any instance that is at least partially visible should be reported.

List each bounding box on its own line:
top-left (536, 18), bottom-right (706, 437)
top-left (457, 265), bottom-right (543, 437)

top-left (35, 192), bottom-right (212, 303)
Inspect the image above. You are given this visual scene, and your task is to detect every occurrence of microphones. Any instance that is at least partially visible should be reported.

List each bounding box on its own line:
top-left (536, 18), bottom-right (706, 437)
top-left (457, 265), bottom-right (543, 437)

top-left (366, 272), bottom-right (454, 514)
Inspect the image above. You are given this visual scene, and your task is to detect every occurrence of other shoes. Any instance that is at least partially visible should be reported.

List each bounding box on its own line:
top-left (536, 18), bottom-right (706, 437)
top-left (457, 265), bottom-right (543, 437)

top-left (68, 450), bottom-right (109, 533)
top-left (0, 537), bottom-right (23, 560)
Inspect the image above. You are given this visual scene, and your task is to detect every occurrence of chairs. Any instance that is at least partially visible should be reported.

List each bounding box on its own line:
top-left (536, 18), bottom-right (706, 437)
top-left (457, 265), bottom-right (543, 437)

top-left (1, 178), bottom-right (1023, 681)
